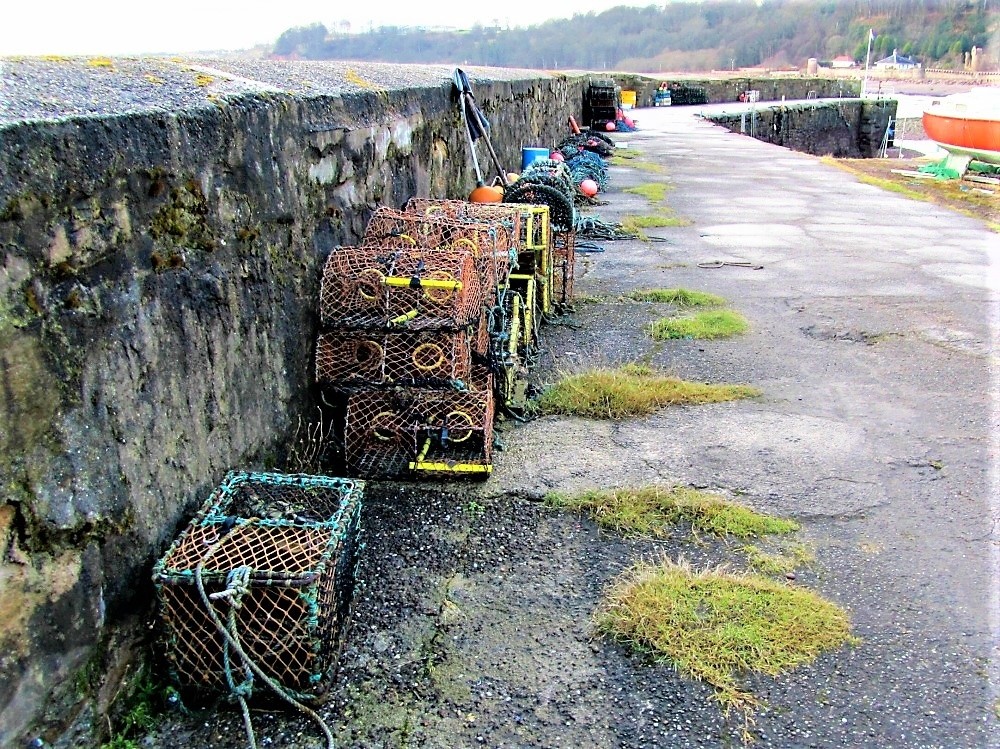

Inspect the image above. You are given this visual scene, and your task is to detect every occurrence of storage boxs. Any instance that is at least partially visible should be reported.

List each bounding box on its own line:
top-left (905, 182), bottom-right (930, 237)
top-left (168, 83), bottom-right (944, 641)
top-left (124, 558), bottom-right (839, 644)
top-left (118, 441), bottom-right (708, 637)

top-left (621, 91), bottom-right (636, 109)
top-left (655, 90), bottom-right (671, 107)
top-left (744, 91), bottom-right (759, 102)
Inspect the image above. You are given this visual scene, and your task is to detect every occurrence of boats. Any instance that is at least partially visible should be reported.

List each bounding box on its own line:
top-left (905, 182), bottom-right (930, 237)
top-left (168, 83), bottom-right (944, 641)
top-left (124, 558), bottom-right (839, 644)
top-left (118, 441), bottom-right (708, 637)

top-left (921, 87), bottom-right (1000, 166)
